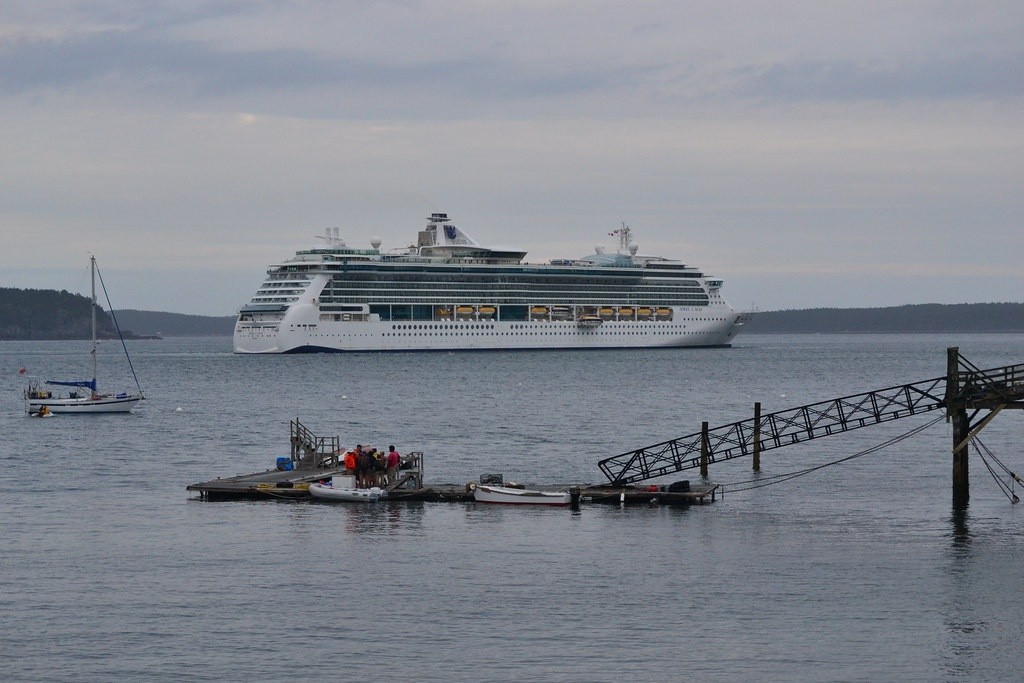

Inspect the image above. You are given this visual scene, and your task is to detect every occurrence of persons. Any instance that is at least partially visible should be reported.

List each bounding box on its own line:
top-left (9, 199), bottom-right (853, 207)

top-left (344, 445), bottom-right (400, 488)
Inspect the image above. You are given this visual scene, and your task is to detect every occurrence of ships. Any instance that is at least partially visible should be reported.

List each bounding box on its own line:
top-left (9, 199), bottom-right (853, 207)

top-left (232, 214), bottom-right (746, 354)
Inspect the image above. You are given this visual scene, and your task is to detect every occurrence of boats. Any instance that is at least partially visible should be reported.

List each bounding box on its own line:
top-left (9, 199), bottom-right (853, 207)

top-left (638, 310), bottom-right (651, 317)
top-left (580, 314), bottom-right (604, 327)
top-left (531, 307), bottom-right (546, 314)
top-left (466, 481), bottom-right (583, 511)
top-left (656, 309), bottom-right (671, 317)
top-left (599, 309), bottom-right (614, 316)
top-left (307, 481), bottom-right (389, 503)
top-left (479, 308), bottom-right (495, 315)
top-left (618, 308), bottom-right (634, 317)
top-left (455, 307), bottom-right (474, 315)
top-left (31, 411), bottom-right (54, 418)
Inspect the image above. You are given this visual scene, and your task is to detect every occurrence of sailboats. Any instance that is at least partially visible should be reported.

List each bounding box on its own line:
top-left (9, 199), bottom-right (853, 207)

top-left (24, 256), bottom-right (146, 414)
